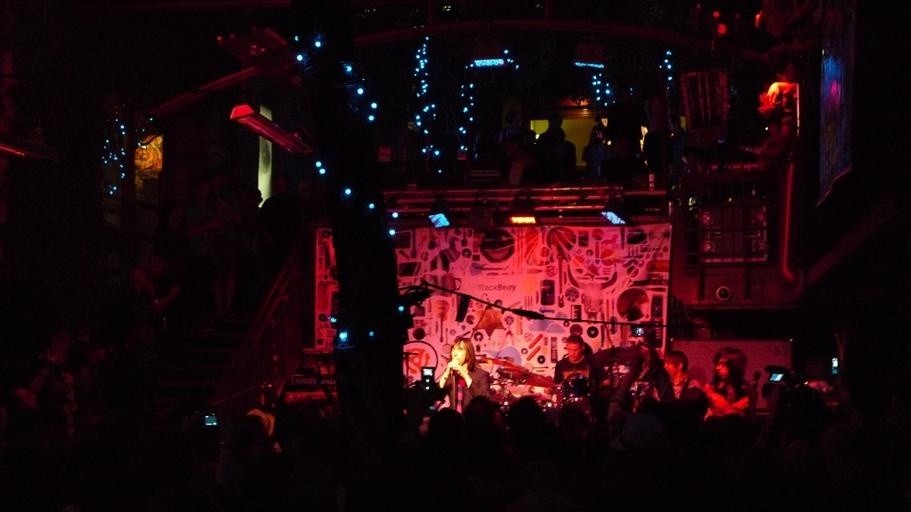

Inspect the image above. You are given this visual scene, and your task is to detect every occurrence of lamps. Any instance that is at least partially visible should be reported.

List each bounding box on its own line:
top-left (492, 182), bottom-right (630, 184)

top-left (424, 190), bottom-right (637, 230)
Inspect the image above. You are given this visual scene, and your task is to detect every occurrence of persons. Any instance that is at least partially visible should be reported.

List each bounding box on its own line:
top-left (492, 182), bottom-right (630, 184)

top-left (2, 108), bottom-right (911, 510)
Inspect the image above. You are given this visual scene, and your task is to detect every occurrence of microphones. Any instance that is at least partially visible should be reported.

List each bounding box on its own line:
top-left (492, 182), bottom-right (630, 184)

top-left (450, 359), bottom-right (457, 373)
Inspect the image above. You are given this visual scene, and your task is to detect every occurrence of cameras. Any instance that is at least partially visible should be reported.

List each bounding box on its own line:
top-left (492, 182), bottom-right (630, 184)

top-left (203, 414), bottom-right (220, 427)
top-left (768, 372), bottom-right (784, 382)
top-left (632, 326), bottom-right (646, 337)
